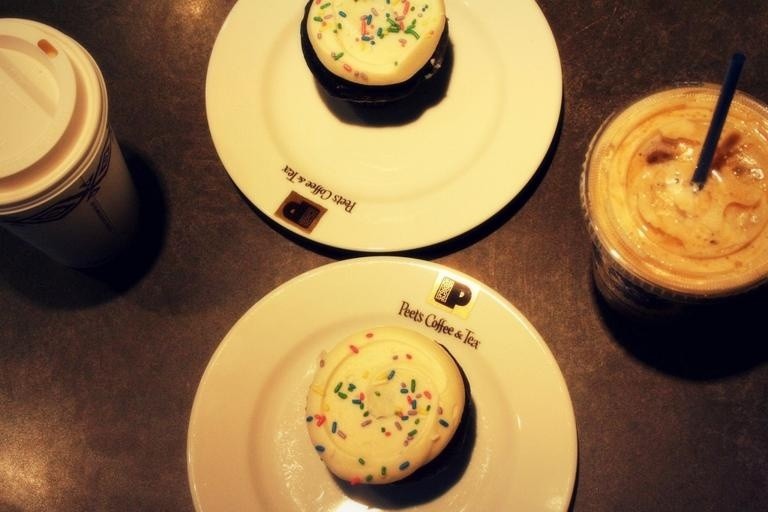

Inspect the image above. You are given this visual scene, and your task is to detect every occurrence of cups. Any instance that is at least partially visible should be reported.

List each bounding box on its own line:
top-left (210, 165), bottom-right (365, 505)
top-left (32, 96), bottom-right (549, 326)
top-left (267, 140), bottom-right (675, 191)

top-left (576, 80), bottom-right (764, 326)
top-left (1, 19), bottom-right (144, 272)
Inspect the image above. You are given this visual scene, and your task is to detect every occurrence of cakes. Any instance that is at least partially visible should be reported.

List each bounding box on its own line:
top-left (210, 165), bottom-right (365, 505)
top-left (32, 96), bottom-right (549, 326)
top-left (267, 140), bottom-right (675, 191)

top-left (303, 326), bottom-right (467, 488)
top-left (305, 0), bottom-right (445, 85)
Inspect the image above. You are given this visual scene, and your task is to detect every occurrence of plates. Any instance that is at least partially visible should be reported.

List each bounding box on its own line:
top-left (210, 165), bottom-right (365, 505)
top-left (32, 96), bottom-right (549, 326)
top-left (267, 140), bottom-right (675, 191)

top-left (198, 1), bottom-right (565, 254)
top-left (181, 253), bottom-right (581, 512)
